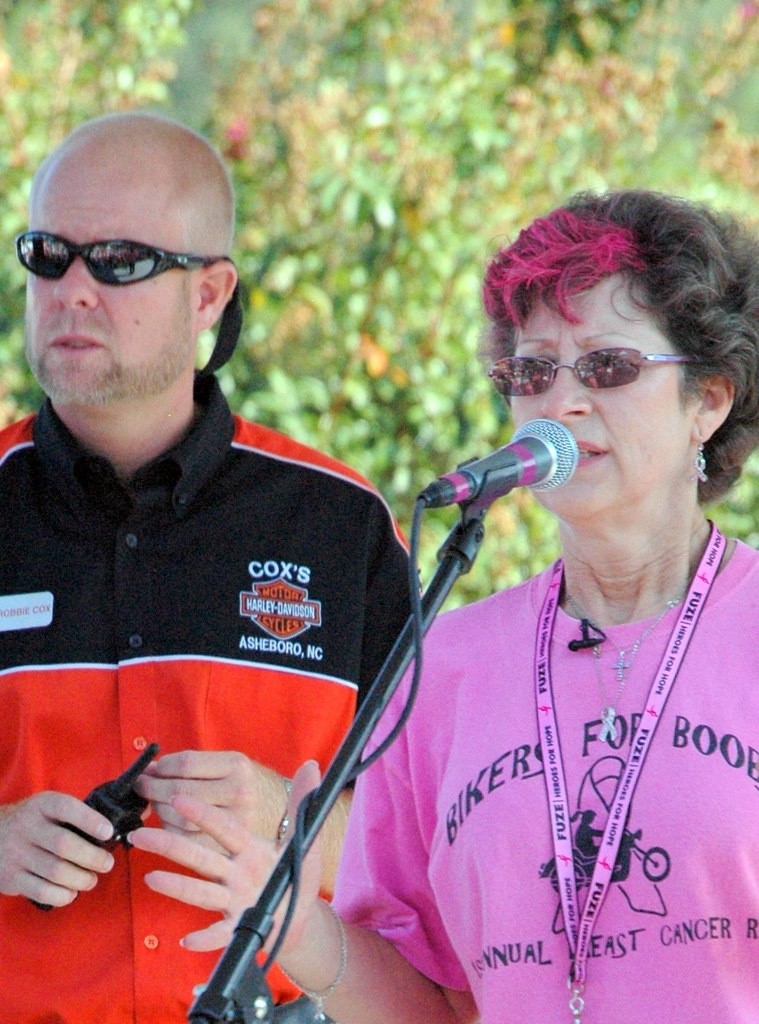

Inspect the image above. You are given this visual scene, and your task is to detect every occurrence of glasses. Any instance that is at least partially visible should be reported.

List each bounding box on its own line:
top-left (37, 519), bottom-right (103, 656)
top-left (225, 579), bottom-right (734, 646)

top-left (16, 231), bottom-right (233, 288)
top-left (488, 348), bottom-right (711, 409)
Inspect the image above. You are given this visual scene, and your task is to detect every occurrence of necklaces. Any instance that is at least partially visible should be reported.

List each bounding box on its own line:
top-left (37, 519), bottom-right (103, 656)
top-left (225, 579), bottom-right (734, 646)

top-left (565, 591), bottom-right (690, 742)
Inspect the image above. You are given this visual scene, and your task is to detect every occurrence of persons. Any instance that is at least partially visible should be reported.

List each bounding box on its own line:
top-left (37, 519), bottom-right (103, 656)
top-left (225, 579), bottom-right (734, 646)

top-left (126, 193), bottom-right (759, 1024)
top-left (0, 110), bottom-right (428, 1023)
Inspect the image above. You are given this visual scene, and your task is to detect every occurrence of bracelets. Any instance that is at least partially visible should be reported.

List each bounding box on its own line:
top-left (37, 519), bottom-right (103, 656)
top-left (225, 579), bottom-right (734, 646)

top-left (278, 777), bottom-right (292, 842)
top-left (277, 903), bottom-right (349, 1019)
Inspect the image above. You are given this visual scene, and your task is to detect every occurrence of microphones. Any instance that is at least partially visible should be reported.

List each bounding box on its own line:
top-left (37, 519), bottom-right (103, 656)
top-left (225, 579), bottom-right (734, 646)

top-left (567, 638), bottom-right (604, 652)
top-left (418, 419), bottom-right (579, 509)
